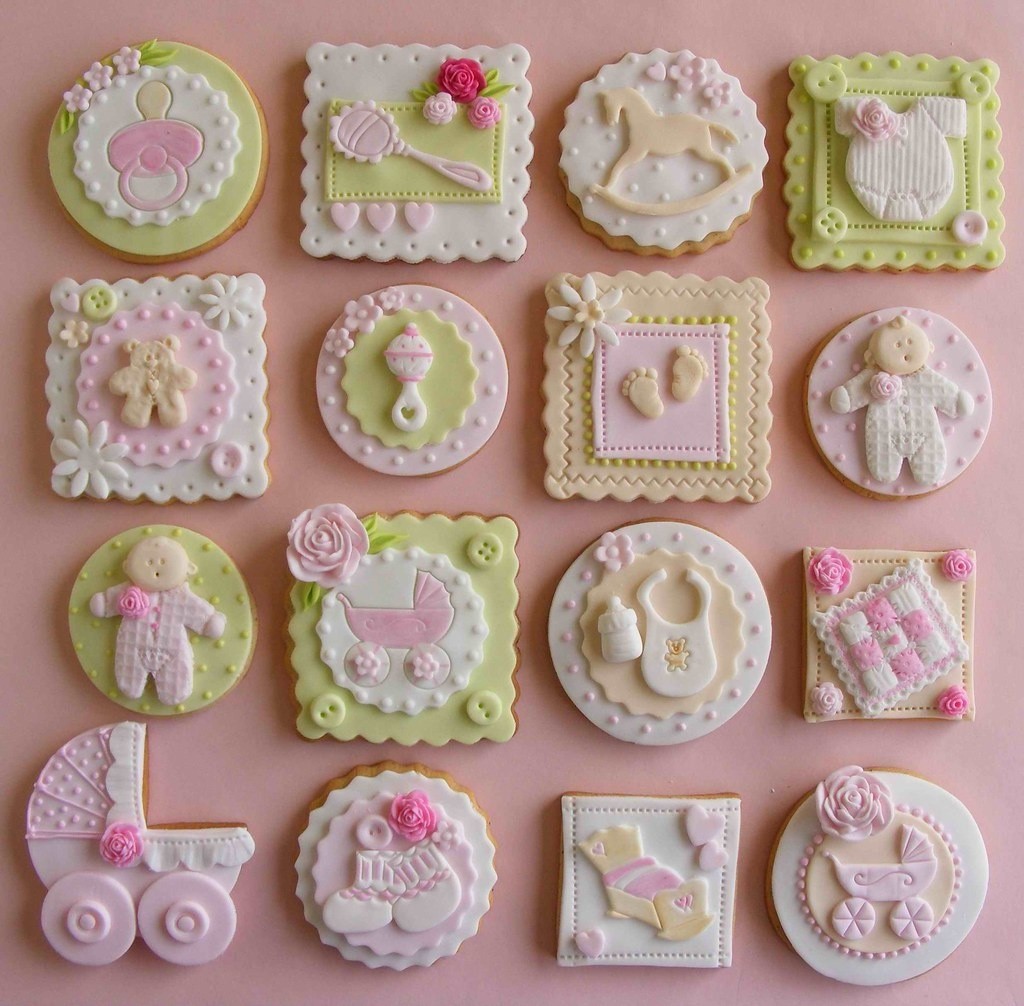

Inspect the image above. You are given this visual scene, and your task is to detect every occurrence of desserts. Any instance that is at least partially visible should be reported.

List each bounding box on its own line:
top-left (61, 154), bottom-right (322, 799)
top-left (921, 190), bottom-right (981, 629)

top-left (27, 39), bottom-right (1005, 987)
